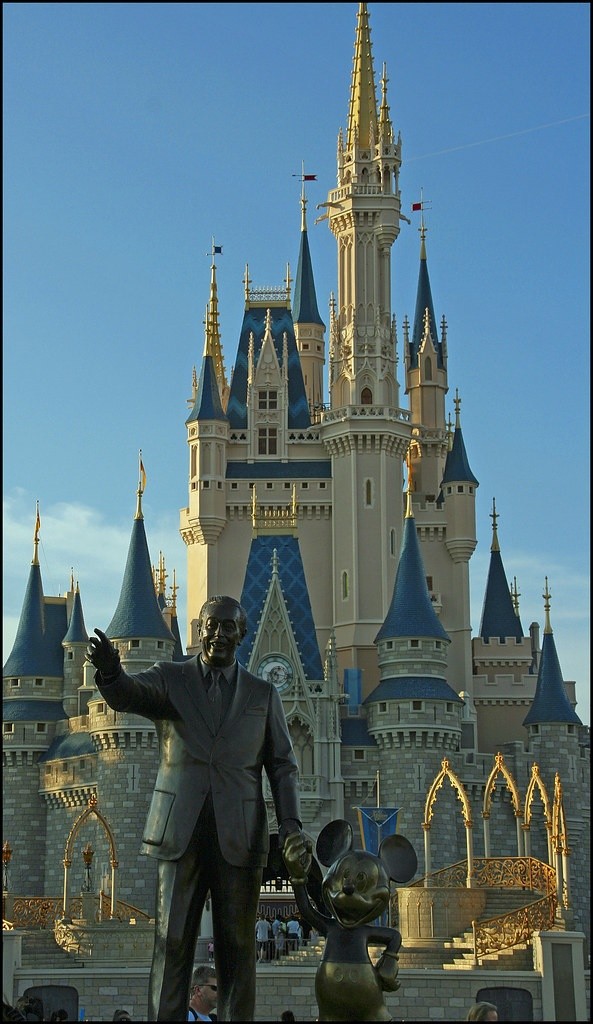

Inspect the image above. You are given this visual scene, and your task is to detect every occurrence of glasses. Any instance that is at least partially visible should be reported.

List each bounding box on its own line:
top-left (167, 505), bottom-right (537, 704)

top-left (193, 985), bottom-right (217, 992)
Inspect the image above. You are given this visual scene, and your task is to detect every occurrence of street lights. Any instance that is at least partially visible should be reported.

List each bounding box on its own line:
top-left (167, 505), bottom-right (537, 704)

top-left (1, 840), bottom-right (15, 895)
top-left (80, 843), bottom-right (95, 898)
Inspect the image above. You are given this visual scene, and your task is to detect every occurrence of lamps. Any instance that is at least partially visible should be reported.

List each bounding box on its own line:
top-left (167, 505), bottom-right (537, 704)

top-left (2, 840), bottom-right (15, 891)
top-left (81, 843), bottom-right (95, 892)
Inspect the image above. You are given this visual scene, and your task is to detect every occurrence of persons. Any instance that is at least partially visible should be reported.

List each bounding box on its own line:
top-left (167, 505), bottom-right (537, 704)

top-left (256, 912), bottom-right (304, 960)
top-left (286, 916), bottom-right (299, 952)
top-left (2, 1002), bottom-right (25, 1021)
top-left (281, 1011), bottom-right (296, 1021)
top-left (467, 1002), bottom-right (498, 1021)
top-left (82, 593), bottom-right (304, 1021)
top-left (208, 940), bottom-right (214, 962)
top-left (272, 914), bottom-right (287, 960)
top-left (51, 1009), bottom-right (68, 1021)
top-left (187, 965), bottom-right (217, 1021)
top-left (309, 926), bottom-right (320, 946)
top-left (255, 913), bottom-right (271, 963)
top-left (114, 1010), bottom-right (132, 1021)
top-left (15, 996), bottom-right (44, 1021)
